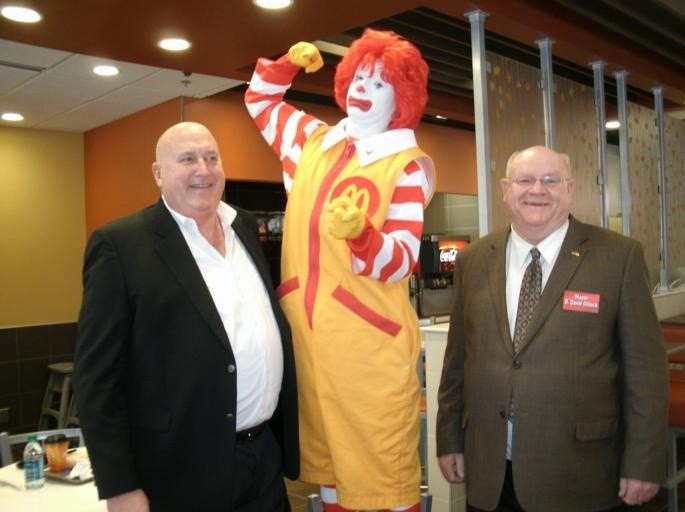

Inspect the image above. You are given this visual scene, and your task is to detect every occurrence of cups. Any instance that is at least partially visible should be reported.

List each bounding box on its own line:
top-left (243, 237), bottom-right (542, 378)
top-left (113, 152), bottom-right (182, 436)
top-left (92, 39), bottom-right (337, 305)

top-left (43, 434), bottom-right (69, 474)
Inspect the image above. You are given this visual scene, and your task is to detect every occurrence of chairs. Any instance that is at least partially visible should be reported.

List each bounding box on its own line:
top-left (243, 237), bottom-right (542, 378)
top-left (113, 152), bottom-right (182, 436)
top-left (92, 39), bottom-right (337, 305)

top-left (658, 313), bottom-right (685, 512)
top-left (0, 427), bottom-right (83, 467)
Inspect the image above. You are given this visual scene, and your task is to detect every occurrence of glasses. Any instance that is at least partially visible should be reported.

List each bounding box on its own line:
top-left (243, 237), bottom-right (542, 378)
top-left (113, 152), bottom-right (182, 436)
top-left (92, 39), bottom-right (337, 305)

top-left (516, 175), bottom-right (562, 187)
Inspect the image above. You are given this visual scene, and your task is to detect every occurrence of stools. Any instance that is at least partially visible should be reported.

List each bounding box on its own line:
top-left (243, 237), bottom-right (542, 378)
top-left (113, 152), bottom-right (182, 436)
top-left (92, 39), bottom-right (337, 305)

top-left (37, 362), bottom-right (75, 430)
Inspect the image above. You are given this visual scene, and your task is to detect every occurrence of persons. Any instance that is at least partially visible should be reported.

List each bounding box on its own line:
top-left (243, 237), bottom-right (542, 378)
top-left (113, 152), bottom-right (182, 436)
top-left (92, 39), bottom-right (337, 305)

top-left (71, 116), bottom-right (303, 511)
top-left (437, 143), bottom-right (667, 510)
top-left (246, 28), bottom-right (437, 510)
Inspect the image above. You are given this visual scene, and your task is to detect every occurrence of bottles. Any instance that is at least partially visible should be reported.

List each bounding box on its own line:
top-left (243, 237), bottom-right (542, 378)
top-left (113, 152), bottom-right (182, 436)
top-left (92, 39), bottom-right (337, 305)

top-left (22, 433), bottom-right (45, 490)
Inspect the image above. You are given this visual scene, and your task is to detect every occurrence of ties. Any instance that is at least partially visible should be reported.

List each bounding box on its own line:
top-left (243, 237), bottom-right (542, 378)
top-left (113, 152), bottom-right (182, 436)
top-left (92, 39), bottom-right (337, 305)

top-left (508, 248), bottom-right (542, 425)
